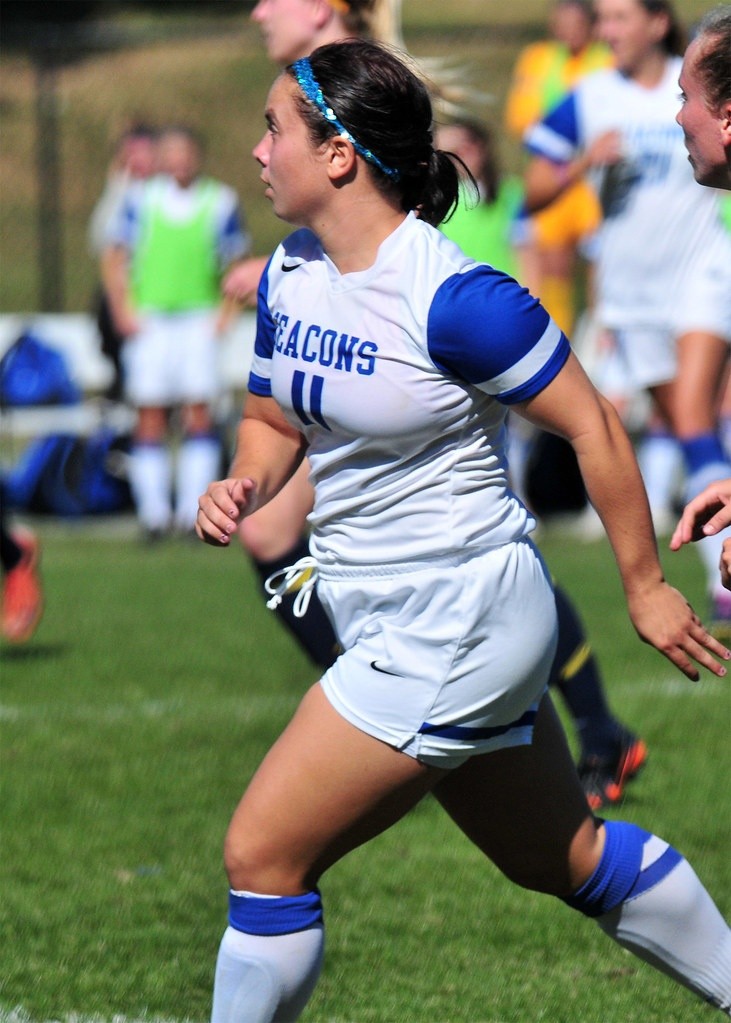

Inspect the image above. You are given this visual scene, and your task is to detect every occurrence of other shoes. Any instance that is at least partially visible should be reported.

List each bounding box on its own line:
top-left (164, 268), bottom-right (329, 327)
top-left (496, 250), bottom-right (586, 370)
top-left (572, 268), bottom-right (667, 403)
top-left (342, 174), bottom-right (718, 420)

top-left (0, 525), bottom-right (42, 645)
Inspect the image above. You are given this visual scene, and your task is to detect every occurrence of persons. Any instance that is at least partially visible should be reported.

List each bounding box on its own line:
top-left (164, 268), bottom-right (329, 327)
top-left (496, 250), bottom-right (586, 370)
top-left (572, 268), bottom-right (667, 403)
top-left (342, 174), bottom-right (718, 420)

top-left (195, 46), bottom-right (731, 1021)
top-left (675, 18), bottom-right (730, 612)
top-left (668, 478), bottom-right (731, 590)
top-left (0, 513), bottom-right (43, 643)
top-left (91, 120), bottom-right (245, 543)
top-left (434, 0), bottom-right (723, 458)
top-left (220, 0), bottom-right (647, 810)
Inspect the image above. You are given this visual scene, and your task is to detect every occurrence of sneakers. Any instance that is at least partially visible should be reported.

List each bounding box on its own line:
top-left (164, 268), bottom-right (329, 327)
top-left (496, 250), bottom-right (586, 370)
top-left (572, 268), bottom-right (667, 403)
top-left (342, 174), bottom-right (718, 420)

top-left (576, 723), bottom-right (646, 811)
